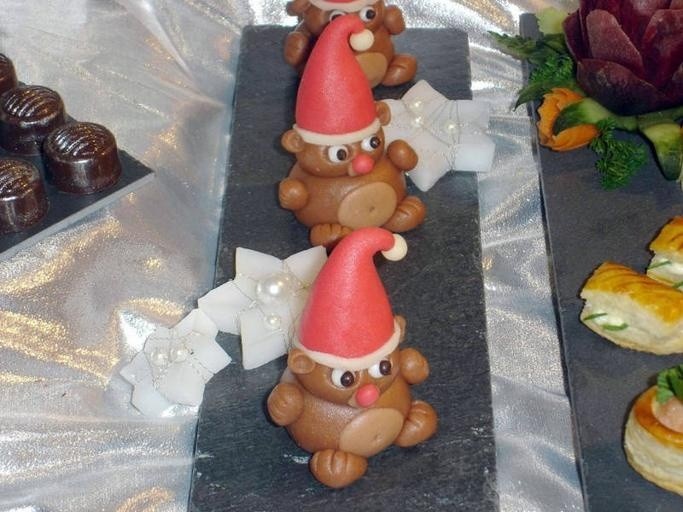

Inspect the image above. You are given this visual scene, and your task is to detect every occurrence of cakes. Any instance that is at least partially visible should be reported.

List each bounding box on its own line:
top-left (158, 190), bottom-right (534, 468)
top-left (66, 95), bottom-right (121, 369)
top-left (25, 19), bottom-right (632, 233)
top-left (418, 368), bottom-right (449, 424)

top-left (0, 55), bottom-right (120, 235)
top-left (577, 262), bottom-right (683, 356)
top-left (645, 216), bottom-right (683, 294)
top-left (278, 19), bottom-right (425, 247)
top-left (267, 229), bottom-right (437, 487)
top-left (622, 367), bottom-right (682, 494)
top-left (281, 1), bottom-right (417, 89)
